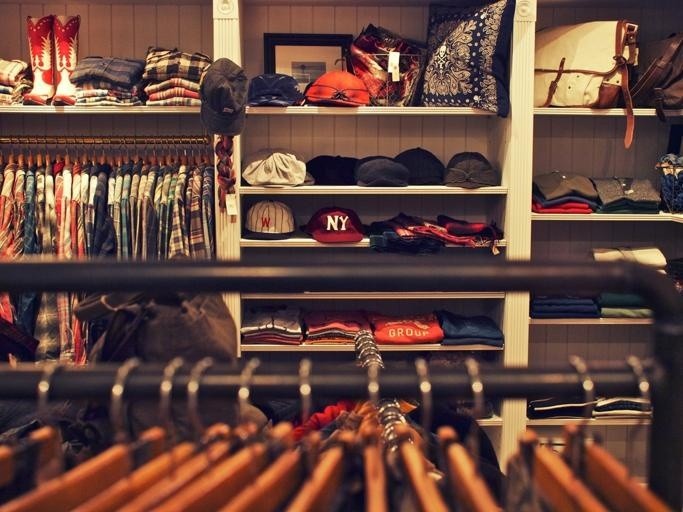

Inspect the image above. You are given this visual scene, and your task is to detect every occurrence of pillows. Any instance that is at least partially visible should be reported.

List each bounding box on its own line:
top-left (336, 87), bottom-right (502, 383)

top-left (416, 0), bottom-right (515, 117)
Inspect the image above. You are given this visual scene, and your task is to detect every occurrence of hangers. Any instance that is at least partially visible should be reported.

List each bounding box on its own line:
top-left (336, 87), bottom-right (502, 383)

top-left (0, 132), bottom-right (210, 168)
top-left (1, 359), bottom-right (673, 510)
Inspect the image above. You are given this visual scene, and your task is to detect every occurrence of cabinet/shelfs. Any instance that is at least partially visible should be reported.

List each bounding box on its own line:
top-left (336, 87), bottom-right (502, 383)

top-left (0, 0), bottom-right (681, 473)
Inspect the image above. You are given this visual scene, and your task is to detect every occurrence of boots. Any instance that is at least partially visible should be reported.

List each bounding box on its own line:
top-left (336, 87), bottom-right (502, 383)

top-left (53, 14), bottom-right (80, 107)
top-left (24, 15), bottom-right (55, 106)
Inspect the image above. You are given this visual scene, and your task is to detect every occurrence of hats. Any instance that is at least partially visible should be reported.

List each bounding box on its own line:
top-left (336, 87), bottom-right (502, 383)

top-left (247, 72), bottom-right (303, 108)
top-left (243, 198), bottom-right (295, 241)
top-left (305, 147), bottom-right (501, 189)
top-left (201, 58), bottom-right (248, 137)
top-left (306, 70), bottom-right (371, 106)
top-left (301, 207), bottom-right (364, 244)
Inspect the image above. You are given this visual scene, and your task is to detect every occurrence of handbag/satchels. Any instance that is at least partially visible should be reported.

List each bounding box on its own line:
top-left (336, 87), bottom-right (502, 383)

top-left (534, 18), bottom-right (683, 110)
top-left (72, 285), bottom-right (241, 432)
top-left (348, 23), bottom-right (429, 107)
